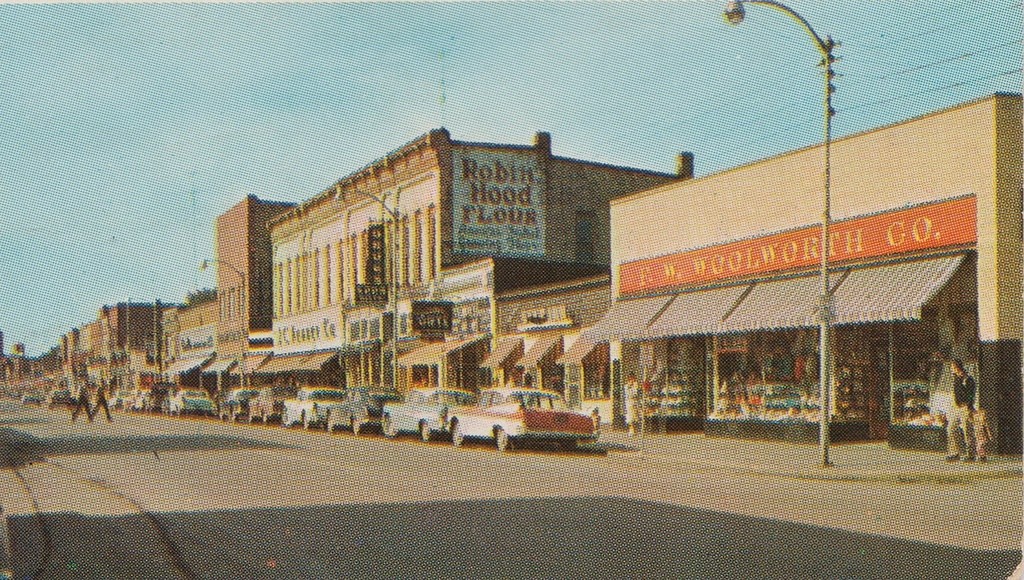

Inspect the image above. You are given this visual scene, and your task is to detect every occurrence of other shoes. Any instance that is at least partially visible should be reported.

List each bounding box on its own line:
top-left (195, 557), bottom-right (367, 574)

top-left (963, 454), bottom-right (975, 461)
top-left (945, 453), bottom-right (959, 462)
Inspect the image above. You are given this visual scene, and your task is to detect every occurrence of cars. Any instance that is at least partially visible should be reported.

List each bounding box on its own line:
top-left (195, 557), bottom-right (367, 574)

top-left (442, 387), bottom-right (597, 453)
top-left (380, 385), bottom-right (478, 443)
top-left (20, 374), bottom-right (404, 435)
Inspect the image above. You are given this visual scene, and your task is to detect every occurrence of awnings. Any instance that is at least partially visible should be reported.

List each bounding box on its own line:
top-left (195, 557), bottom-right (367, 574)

top-left (163, 350), bottom-right (334, 377)
top-left (391, 247), bottom-right (980, 368)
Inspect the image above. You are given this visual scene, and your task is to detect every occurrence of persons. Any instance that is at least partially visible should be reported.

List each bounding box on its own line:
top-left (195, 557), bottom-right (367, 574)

top-left (88, 379), bottom-right (114, 423)
top-left (550, 373), bottom-right (565, 401)
top-left (975, 405), bottom-right (995, 462)
top-left (72, 380), bottom-right (92, 423)
top-left (624, 373), bottom-right (639, 435)
top-left (943, 359), bottom-right (977, 464)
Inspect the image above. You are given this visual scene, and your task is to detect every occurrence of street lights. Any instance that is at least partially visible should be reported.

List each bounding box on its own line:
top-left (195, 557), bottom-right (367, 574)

top-left (722, 0), bottom-right (832, 469)
top-left (331, 183), bottom-right (401, 390)
top-left (200, 259), bottom-right (246, 387)
